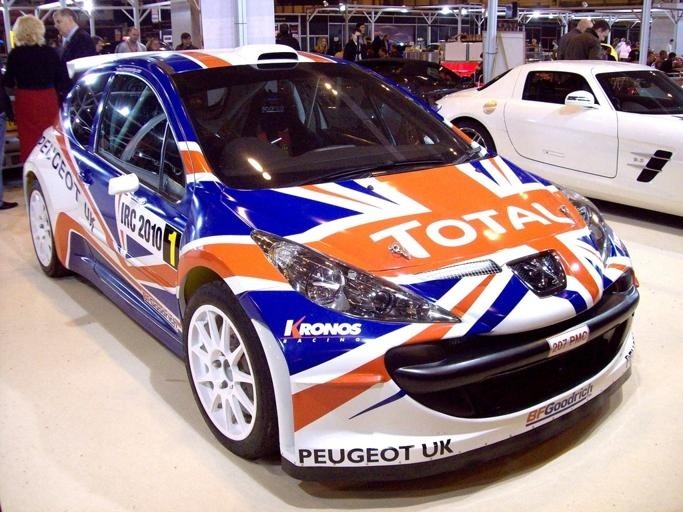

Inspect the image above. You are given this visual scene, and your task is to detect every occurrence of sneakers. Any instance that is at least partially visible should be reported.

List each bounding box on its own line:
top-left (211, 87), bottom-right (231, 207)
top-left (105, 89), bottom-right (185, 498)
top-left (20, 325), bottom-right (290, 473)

top-left (0, 201), bottom-right (18, 210)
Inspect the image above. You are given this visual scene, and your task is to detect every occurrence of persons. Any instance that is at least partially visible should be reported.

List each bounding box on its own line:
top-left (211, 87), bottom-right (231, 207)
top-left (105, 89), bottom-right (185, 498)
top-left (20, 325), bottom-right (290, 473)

top-left (45, 24), bottom-right (199, 62)
top-left (276, 21), bottom-right (402, 62)
top-left (52, 8), bottom-right (97, 105)
top-left (529, 18), bottom-right (683, 77)
top-left (3, 15), bottom-right (64, 163)
top-left (0, 69), bottom-right (18, 209)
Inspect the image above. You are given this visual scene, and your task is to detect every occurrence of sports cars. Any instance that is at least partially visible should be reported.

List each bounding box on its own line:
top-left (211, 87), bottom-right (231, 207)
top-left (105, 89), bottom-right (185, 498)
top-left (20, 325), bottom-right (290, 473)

top-left (435, 59), bottom-right (682, 219)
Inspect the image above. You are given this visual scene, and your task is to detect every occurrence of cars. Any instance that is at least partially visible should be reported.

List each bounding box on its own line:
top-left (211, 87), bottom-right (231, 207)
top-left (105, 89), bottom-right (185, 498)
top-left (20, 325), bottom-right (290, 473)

top-left (438, 57), bottom-right (480, 89)
top-left (20, 43), bottom-right (638, 482)
top-left (355, 60), bottom-right (473, 108)
top-left (0, 54), bottom-right (23, 170)
top-left (374, 24), bottom-right (411, 47)
top-left (599, 43), bottom-right (619, 61)
